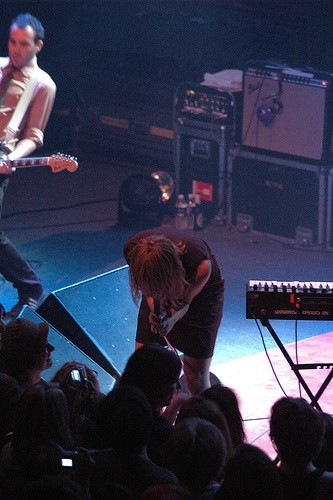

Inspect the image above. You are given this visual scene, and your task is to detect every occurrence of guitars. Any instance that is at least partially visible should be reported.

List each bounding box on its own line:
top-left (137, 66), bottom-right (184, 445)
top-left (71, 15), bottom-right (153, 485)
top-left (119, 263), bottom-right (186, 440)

top-left (0, 141), bottom-right (78, 173)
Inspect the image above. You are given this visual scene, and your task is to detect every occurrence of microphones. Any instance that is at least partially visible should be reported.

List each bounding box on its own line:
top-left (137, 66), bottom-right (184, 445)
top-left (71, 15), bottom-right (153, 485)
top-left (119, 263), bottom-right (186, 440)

top-left (154, 297), bottom-right (160, 340)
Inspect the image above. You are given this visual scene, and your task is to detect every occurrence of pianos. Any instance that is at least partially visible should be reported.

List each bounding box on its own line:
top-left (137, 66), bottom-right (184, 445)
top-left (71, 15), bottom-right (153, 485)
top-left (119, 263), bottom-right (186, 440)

top-left (246, 279), bottom-right (333, 321)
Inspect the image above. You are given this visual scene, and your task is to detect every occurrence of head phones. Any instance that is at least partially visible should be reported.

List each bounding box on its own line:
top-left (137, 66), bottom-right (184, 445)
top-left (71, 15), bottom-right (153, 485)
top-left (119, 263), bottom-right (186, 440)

top-left (256, 99), bottom-right (282, 127)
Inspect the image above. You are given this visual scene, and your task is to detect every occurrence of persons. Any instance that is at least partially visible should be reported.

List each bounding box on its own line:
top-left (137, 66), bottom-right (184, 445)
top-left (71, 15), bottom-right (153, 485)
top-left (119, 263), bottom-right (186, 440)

top-left (0, 13), bottom-right (56, 205)
top-left (123, 231), bottom-right (225, 398)
top-left (1, 318), bottom-right (333, 500)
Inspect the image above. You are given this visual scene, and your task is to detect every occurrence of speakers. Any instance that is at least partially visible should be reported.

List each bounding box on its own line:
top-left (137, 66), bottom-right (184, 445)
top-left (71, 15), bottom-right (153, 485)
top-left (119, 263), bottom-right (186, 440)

top-left (240, 68), bottom-right (333, 166)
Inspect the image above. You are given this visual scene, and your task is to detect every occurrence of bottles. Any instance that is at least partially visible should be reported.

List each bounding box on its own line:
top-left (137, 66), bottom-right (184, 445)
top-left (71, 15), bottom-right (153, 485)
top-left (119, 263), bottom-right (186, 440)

top-left (186, 194), bottom-right (195, 231)
top-left (175, 194), bottom-right (187, 230)
top-left (194, 192), bottom-right (204, 230)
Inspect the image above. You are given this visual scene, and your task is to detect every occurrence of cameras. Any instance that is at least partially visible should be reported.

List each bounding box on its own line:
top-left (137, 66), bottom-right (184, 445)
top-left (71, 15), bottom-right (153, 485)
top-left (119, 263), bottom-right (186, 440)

top-left (57, 450), bottom-right (76, 470)
top-left (68, 364), bottom-right (88, 386)
